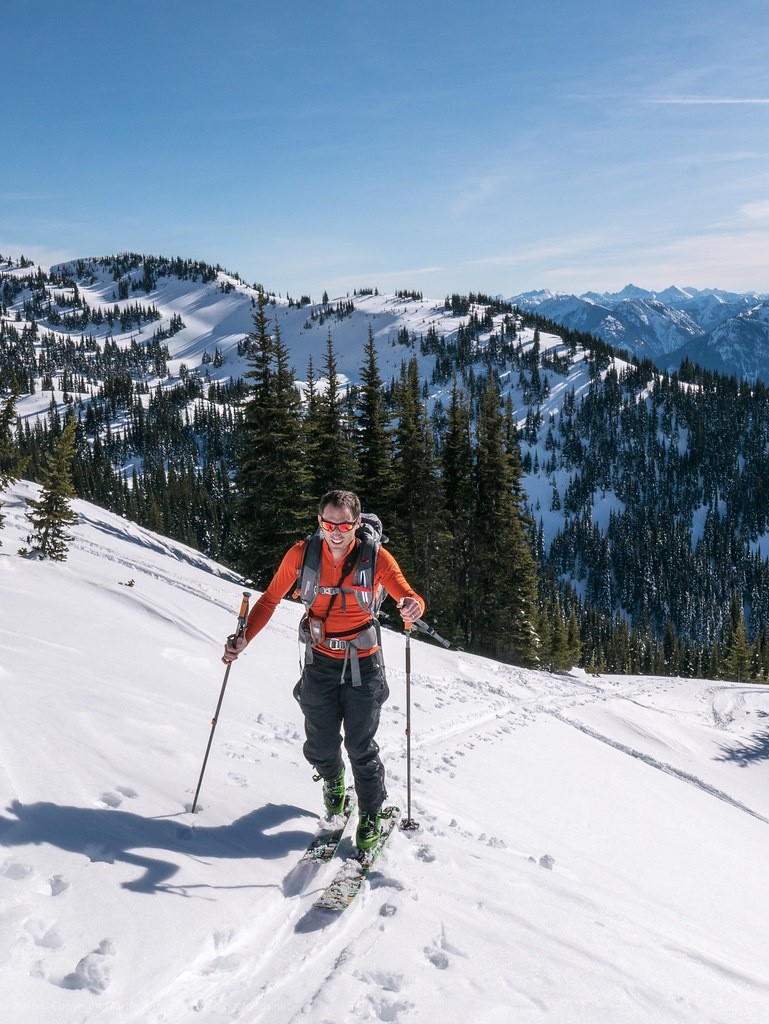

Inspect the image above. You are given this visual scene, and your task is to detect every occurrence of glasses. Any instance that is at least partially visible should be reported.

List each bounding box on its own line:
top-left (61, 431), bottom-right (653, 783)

top-left (321, 519), bottom-right (359, 533)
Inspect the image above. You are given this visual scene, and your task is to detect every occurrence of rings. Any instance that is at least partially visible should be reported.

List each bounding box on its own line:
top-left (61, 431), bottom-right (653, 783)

top-left (411, 614), bottom-right (414, 618)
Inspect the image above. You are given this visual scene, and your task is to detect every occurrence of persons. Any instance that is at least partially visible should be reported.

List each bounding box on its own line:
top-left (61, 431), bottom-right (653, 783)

top-left (221, 491), bottom-right (426, 852)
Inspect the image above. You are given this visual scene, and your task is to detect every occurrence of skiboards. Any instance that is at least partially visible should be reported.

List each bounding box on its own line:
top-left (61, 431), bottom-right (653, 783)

top-left (297, 783), bottom-right (401, 912)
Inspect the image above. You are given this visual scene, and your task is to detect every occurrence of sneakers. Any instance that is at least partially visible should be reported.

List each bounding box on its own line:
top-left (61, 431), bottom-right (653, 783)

top-left (354, 805), bottom-right (382, 848)
top-left (322, 766), bottom-right (346, 815)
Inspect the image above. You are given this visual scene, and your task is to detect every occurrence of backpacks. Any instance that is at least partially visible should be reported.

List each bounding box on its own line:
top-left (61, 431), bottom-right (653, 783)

top-left (302, 513), bottom-right (387, 607)
